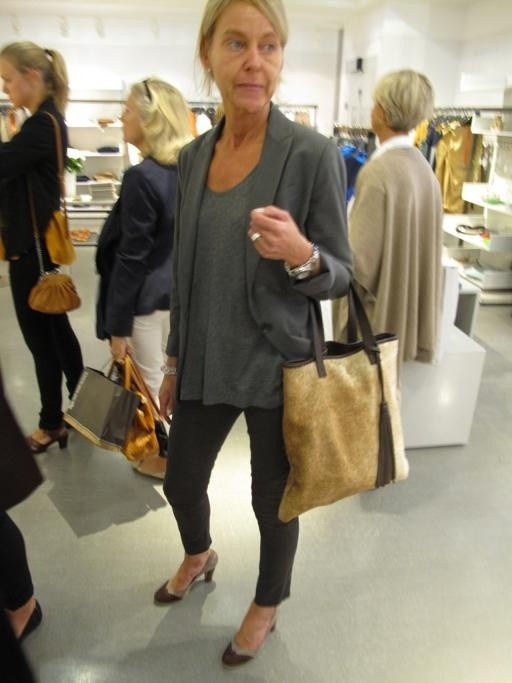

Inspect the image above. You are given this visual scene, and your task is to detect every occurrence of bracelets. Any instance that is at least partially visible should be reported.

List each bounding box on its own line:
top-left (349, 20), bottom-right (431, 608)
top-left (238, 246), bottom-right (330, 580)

top-left (160, 363), bottom-right (180, 375)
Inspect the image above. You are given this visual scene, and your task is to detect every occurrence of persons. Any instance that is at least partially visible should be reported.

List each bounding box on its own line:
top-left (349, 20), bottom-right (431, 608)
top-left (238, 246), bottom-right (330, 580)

top-left (96, 74), bottom-right (198, 481)
top-left (0, 39), bottom-right (87, 458)
top-left (1, 364), bottom-right (48, 682)
top-left (151, 0), bottom-right (357, 669)
top-left (332, 68), bottom-right (443, 409)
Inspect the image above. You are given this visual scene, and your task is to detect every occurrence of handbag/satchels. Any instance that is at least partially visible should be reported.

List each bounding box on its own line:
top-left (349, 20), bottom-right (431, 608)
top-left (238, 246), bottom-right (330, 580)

top-left (62, 352), bottom-right (168, 461)
top-left (46, 210), bottom-right (77, 265)
top-left (26, 273), bottom-right (82, 314)
top-left (277, 278), bottom-right (409, 523)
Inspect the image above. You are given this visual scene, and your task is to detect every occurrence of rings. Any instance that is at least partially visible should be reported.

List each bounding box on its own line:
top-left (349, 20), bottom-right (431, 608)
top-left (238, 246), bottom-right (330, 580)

top-left (250, 231), bottom-right (259, 243)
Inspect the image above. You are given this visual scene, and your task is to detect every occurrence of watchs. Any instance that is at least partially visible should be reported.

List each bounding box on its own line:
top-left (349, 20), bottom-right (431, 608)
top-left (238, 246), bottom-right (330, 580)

top-left (284, 241), bottom-right (321, 281)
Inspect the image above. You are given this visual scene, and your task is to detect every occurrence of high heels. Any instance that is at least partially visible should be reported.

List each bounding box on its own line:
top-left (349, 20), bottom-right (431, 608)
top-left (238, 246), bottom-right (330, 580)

top-left (26, 429), bottom-right (68, 453)
top-left (154, 549), bottom-right (218, 602)
top-left (222, 602), bottom-right (278, 666)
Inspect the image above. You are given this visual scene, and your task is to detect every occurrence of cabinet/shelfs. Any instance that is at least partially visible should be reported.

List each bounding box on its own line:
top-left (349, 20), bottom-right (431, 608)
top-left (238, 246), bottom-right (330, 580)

top-left (61, 120), bottom-right (132, 248)
top-left (382, 249), bottom-right (489, 450)
top-left (439, 105), bottom-right (511, 308)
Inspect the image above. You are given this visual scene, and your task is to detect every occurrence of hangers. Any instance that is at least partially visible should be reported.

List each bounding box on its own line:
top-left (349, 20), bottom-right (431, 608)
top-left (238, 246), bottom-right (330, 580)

top-left (329, 122), bottom-right (380, 162)
top-left (277, 104), bottom-right (312, 118)
top-left (427, 103), bottom-right (480, 130)
top-left (190, 104), bottom-right (218, 127)
